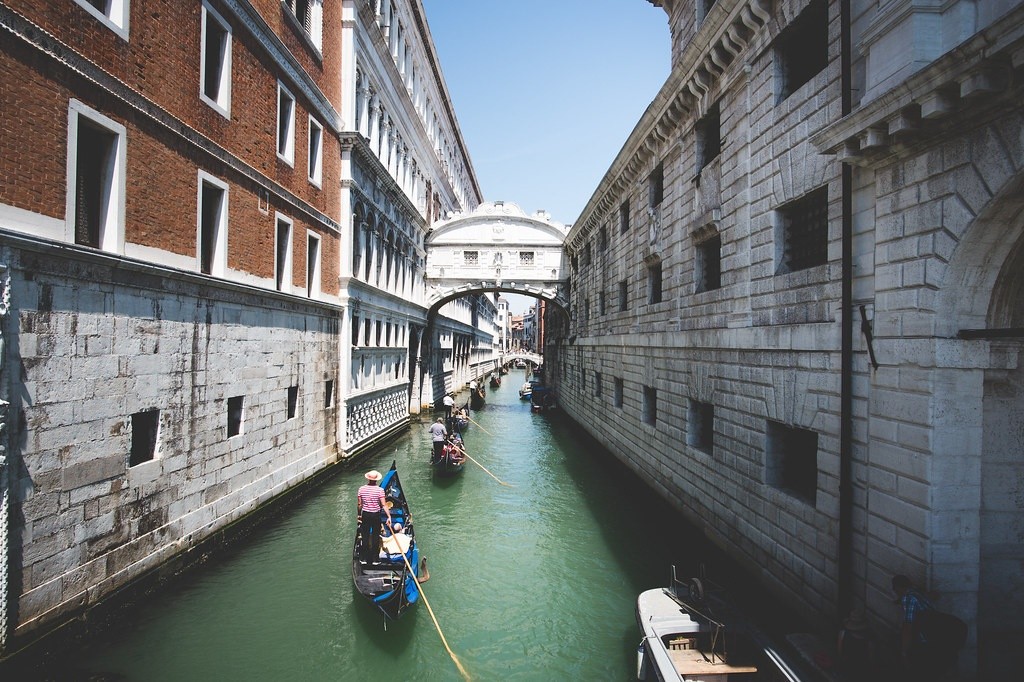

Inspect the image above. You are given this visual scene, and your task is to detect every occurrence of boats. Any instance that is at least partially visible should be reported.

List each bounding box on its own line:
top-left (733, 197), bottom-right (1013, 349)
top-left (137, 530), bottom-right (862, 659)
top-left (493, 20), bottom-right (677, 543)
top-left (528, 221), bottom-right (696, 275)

top-left (489, 375), bottom-right (501, 388)
top-left (636, 563), bottom-right (800, 682)
top-left (470, 382), bottom-right (485, 408)
top-left (430, 418), bottom-right (466, 476)
top-left (446, 397), bottom-right (470, 431)
top-left (518, 379), bottom-right (557, 413)
top-left (499, 358), bottom-right (526, 375)
top-left (352, 459), bottom-right (419, 624)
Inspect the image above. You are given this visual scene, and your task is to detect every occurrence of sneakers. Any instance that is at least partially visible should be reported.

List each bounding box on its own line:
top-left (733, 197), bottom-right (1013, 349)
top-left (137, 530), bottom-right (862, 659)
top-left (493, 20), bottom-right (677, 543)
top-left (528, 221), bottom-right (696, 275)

top-left (372, 560), bottom-right (381, 565)
top-left (360, 560), bottom-right (367, 564)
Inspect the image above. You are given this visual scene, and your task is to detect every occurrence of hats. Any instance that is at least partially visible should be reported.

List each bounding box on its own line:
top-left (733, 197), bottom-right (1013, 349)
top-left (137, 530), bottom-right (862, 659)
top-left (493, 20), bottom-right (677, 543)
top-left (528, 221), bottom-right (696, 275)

top-left (365, 470), bottom-right (382, 481)
top-left (437, 417), bottom-right (443, 421)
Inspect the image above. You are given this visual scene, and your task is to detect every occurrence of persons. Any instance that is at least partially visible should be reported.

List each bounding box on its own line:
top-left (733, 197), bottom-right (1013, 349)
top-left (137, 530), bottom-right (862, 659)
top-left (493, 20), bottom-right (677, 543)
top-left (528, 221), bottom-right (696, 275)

top-left (449, 450), bottom-right (463, 463)
top-left (480, 386), bottom-right (485, 392)
top-left (357, 470), bottom-right (391, 565)
top-left (478, 380), bottom-right (480, 384)
top-left (385, 523), bottom-right (410, 554)
top-left (428, 417), bottom-right (447, 462)
top-left (491, 371), bottom-right (495, 379)
top-left (470, 379), bottom-right (478, 396)
top-left (442, 441), bottom-right (451, 455)
top-left (455, 407), bottom-right (461, 415)
top-left (452, 433), bottom-right (460, 445)
top-left (443, 392), bottom-right (455, 419)
